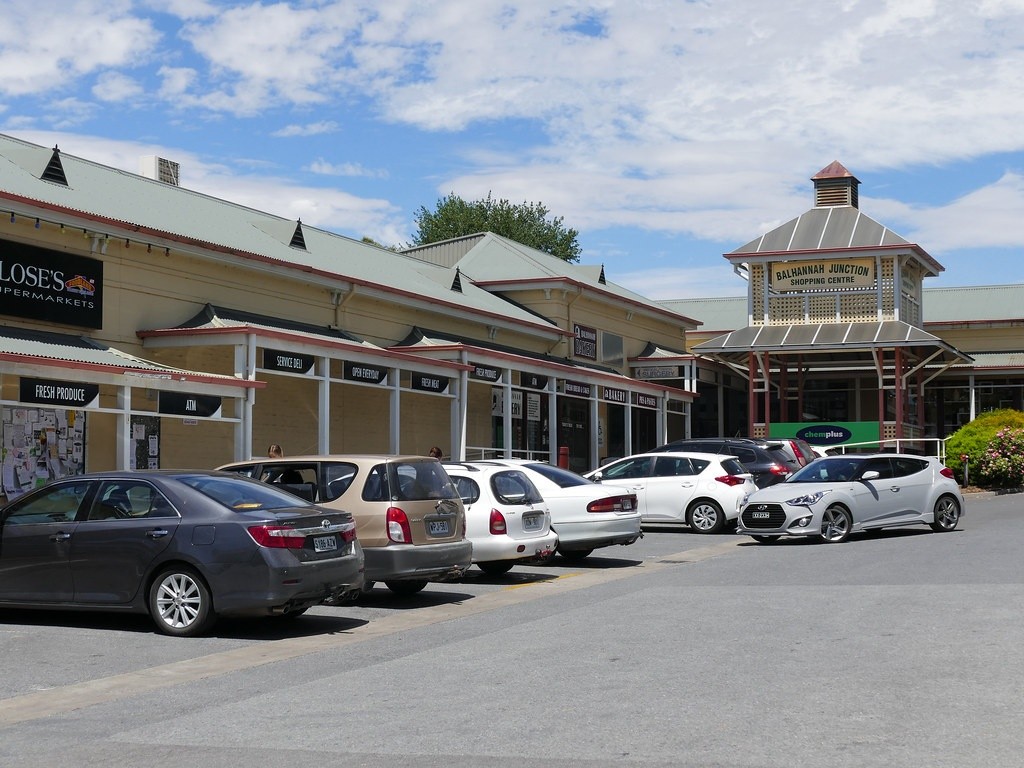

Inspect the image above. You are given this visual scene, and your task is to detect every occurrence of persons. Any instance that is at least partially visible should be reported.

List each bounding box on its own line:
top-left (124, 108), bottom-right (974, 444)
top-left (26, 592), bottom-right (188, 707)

top-left (261, 444), bottom-right (285, 483)
top-left (429, 447), bottom-right (443, 461)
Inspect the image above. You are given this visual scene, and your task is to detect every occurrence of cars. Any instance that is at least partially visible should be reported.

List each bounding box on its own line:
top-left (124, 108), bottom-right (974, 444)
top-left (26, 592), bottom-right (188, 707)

top-left (0, 468), bottom-right (368, 636)
top-left (580, 452), bottom-right (761, 534)
top-left (739, 452), bottom-right (966, 544)
top-left (639, 435), bottom-right (847, 492)
top-left (475, 457), bottom-right (644, 564)
top-left (326, 461), bottom-right (560, 577)
top-left (190, 455), bottom-right (474, 602)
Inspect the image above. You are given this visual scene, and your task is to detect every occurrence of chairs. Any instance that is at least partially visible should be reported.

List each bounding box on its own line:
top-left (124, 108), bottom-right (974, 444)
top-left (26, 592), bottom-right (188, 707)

top-left (110, 488), bottom-right (133, 514)
top-left (280, 471), bottom-right (304, 491)
